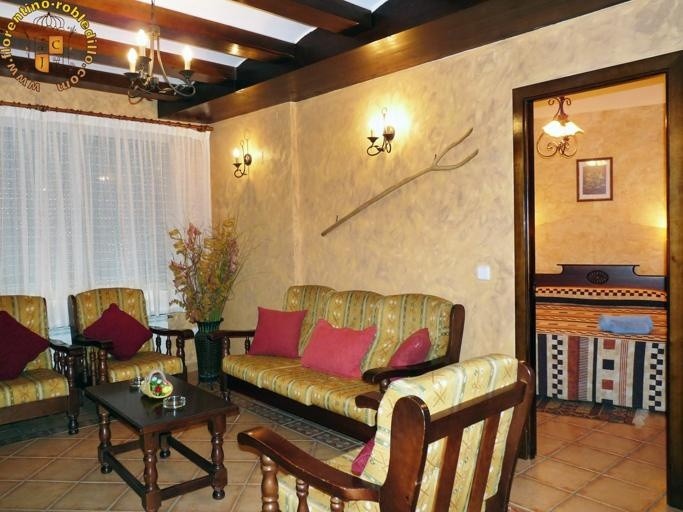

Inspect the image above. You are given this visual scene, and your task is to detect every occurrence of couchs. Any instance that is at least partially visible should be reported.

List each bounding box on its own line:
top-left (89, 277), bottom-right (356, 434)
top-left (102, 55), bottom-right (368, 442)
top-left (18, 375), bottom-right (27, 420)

top-left (208, 285), bottom-right (465, 443)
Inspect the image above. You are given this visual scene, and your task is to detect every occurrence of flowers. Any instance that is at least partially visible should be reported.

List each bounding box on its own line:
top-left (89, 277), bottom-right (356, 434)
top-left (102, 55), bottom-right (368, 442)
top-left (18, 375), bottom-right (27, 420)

top-left (163, 196), bottom-right (257, 323)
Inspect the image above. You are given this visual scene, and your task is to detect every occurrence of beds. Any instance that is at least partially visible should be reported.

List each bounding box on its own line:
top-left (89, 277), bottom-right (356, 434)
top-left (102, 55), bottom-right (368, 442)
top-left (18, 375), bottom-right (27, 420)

top-left (535, 264), bottom-right (666, 412)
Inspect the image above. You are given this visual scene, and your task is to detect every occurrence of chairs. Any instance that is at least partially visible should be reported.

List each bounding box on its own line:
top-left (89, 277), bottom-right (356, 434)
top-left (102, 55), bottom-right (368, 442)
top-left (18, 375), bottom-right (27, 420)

top-left (237, 354), bottom-right (535, 512)
top-left (0, 295), bottom-right (86, 437)
top-left (68, 288), bottom-right (196, 385)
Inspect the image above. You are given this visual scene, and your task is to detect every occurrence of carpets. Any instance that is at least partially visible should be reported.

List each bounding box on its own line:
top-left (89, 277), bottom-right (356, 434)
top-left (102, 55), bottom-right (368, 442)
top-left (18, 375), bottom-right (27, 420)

top-left (536, 397), bottom-right (649, 426)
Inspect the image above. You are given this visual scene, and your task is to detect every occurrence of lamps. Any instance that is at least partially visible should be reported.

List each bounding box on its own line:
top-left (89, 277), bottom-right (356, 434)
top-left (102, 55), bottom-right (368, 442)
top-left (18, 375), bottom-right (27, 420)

top-left (232, 140), bottom-right (252, 178)
top-left (536, 93), bottom-right (584, 158)
top-left (124, 0), bottom-right (196, 104)
top-left (367, 108), bottom-right (395, 156)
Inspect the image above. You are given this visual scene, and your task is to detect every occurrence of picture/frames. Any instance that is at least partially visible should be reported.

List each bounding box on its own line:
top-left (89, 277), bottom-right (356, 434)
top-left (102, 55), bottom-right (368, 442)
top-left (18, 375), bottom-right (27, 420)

top-left (576, 157), bottom-right (613, 202)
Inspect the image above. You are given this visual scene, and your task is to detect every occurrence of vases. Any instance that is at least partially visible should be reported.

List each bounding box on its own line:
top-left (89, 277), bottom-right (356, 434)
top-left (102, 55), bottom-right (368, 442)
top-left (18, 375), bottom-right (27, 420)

top-left (194, 318), bottom-right (224, 382)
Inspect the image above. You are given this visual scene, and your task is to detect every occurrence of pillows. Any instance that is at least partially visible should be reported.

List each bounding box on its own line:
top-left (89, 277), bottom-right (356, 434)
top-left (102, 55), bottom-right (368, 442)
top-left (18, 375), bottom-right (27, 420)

top-left (83, 303), bottom-right (153, 359)
top-left (387, 328), bottom-right (432, 381)
top-left (248, 307), bottom-right (309, 358)
top-left (0, 311), bottom-right (50, 380)
top-left (352, 439), bottom-right (375, 476)
top-left (300, 319), bottom-right (377, 378)
